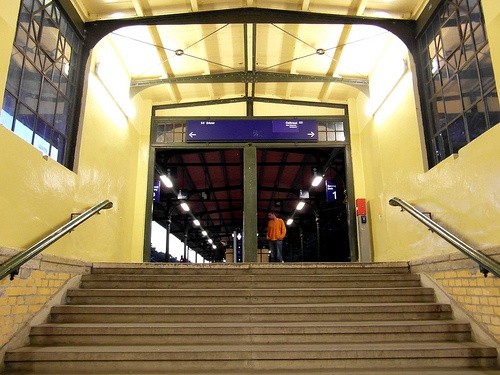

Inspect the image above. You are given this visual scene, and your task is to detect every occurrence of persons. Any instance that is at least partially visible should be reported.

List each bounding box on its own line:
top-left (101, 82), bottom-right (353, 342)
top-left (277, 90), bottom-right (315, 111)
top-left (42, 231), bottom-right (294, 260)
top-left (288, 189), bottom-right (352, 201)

top-left (265, 211), bottom-right (287, 263)
